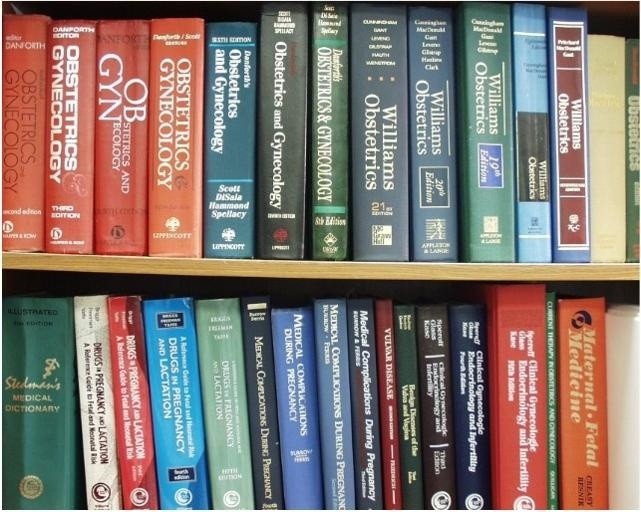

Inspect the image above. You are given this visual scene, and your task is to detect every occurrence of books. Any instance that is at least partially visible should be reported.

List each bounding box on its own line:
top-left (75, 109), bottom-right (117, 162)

top-left (2, 295), bottom-right (78, 510)
top-left (353, 1), bottom-right (409, 262)
top-left (511, 4), bottom-right (553, 263)
top-left (312, 297), bottom-right (356, 511)
top-left (193, 298), bottom-right (256, 510)
top-left (605, 299), bottom-right (639, 510)
top-left (626, 38), bottom-right (639, 264)
top-left (239, 296), bottom-right (284, 509)
top-left (44, 19), bottom-right (94, 255)
top-left (587, 33), bottom-right (626, 264)
top-left (556, 293), bottom-right (606, 510)
top-left (203, 22), bottom-right (258, 259)
top-left (373, 301), bottom-right (403, 511)
top-left (270, 305), bottom-right (326, 511)
top-left (92, 19), bottom-right (149, 257)
top-left (139, 298), bottom-right (212, 510)
top-left (548, 5), bottom-right (592, 262)
top-left (346, 298), bottom-right (385, 510)
top-left (407, 2), bottom-right (459, 262)
top-left (448, 302), bottom-right (490, 510)
top-left (148, 17), bottom-right (205, 258)
top-left (393, 305), bottom-right (426, 510)
top-left (105, 294), bottom-right (169, 510)
top-left (477, 283), bottom-right (547, 510)
top-left (547, 290), bottom-right (556, 510)
top-left (1, 13), bottom-right (51, 253)
top-left (311, 1), bottom-right (351, 261)
top-left (73, 296), bottom-right (122, 511)
top-left (455, 4), bottom-right (514, 263)
top-left (414, 304), bottom-right (457, 510)
top-left (256, 2), bottom-right (307, 259)
